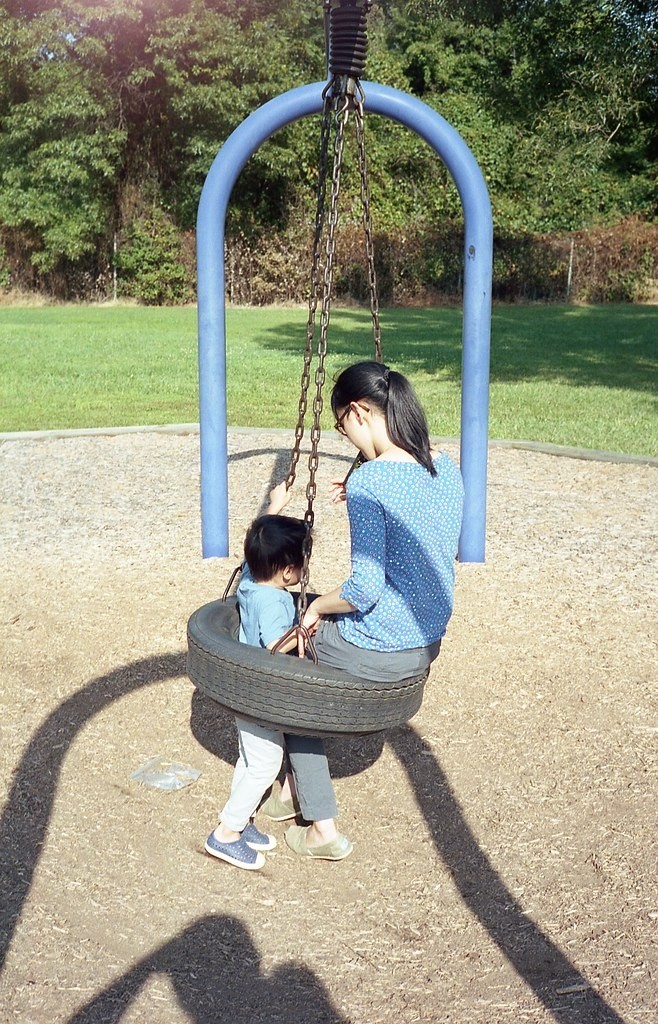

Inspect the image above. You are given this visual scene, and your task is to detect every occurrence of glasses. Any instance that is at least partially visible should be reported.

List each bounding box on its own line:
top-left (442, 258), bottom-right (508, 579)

top-left (334, 403), bottom-right (370, 436)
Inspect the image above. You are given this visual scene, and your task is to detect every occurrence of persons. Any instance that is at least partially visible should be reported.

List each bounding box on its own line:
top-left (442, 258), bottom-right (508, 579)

top-left (262, 362), bottom-right (464, 862)
top-left (205, 481), bottom-right (324, 870)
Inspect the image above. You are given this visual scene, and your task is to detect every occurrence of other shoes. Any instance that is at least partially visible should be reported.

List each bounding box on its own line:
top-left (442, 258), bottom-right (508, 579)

top-left (205, 827), bottom-right (266, 870)
top-left (285, 824), bottom-right (353, 860)
top-left (260, 791), bottom-right (302, 821)
top-left (240, 824), bottom-right (277, 852)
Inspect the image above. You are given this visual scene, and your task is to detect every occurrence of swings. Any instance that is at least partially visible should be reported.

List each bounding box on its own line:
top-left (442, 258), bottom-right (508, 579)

top-left (184, 68), bottom-right (434, 734)
